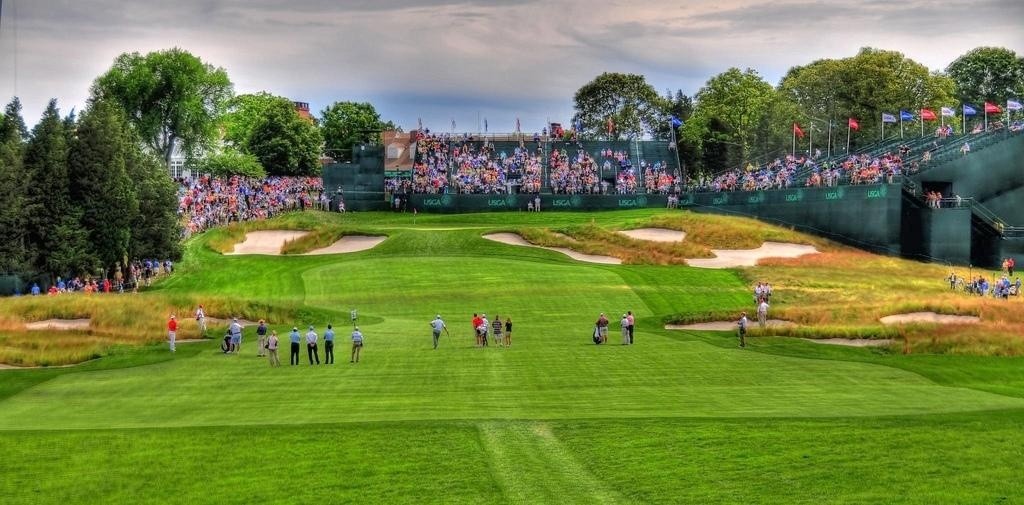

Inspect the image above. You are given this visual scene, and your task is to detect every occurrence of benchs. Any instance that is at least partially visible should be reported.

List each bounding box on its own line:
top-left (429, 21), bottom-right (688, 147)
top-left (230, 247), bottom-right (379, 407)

top-left (407, 137), bottom-right (680, 199)
top-left (703, 123), bottom-right (1019, 186)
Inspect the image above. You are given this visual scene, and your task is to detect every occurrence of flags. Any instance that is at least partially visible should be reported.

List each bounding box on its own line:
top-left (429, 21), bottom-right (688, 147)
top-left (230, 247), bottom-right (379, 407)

top-left (790, 100), bottom-right (1023, 158)
top-left (418, 113), bottom-right (684, 142)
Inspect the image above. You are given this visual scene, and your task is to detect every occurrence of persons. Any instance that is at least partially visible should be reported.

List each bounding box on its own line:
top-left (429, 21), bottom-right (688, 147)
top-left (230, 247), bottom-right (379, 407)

top-left (385, 130), bottom-right (682, 216)
top-left (738, 281), bottom-right (772, 347)
top-left (30, 257), bottom-right (173, 296)
top-left (429, 313), bottom-right (512, 349)
top-left (592, 311), bottom-right (635, 345)
top-left (947, 218), bottom-right (1022, 300)
top-left (171, 174), bottom-right (346, 242)
top-left (167, 304), bottom-right (363, 366)
top-left (682, 130), bottom-right (1013, 209)
